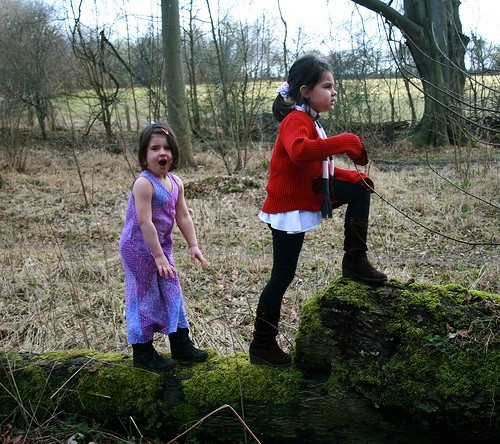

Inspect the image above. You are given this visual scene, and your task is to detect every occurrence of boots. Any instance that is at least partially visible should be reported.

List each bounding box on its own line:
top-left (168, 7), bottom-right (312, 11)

top-left (168, 328), bottom-right (208, 362)
top-left (132, 341), bottom-right (175, 371)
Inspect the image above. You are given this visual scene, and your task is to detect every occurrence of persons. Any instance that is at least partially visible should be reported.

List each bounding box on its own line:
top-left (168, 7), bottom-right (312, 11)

top-left (118, 122), bottom-right (206, 373)
top-left (250, 53), bottom-right (389, 368)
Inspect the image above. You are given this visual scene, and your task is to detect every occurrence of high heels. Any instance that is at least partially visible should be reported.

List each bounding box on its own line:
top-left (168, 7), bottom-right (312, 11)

top-left (342, 253), bottom-right (388, 282)
top-left (248, 341), bottom-right (293, 367)
